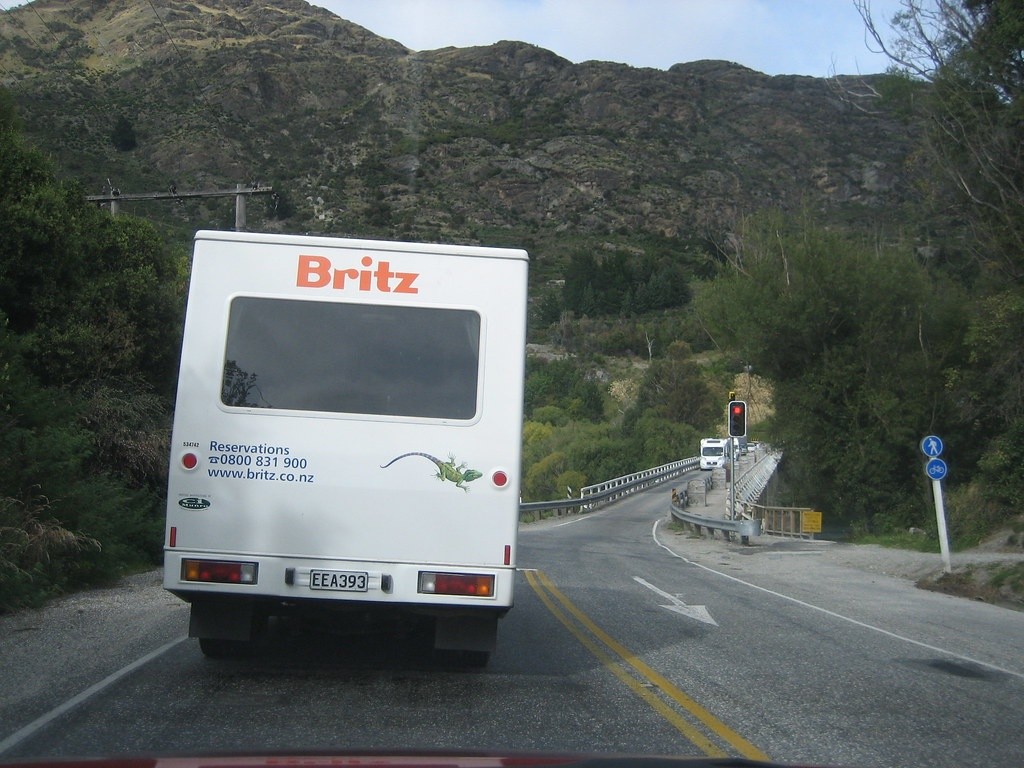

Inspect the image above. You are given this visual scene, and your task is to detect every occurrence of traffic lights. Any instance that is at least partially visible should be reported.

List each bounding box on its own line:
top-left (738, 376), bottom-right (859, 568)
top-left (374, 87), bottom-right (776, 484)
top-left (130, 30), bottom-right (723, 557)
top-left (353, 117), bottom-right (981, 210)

top-left (727, 400), bottom-right (747, 437)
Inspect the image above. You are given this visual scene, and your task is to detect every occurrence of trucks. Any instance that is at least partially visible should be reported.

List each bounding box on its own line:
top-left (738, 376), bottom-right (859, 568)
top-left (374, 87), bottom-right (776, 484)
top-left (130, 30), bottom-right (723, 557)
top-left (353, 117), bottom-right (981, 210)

top-left (699, 437), bottom-right (732, 471)
top-left (159, 228), bottom-right (532, 667)
top-left (729, 436), bottom-right (748, 461)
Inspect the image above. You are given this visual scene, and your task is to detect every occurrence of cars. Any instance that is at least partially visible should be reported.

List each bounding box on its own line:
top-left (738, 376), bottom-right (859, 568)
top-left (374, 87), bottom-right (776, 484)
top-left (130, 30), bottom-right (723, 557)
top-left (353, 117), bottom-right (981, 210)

top-left (747, 440), bottom-right (764, 452)
